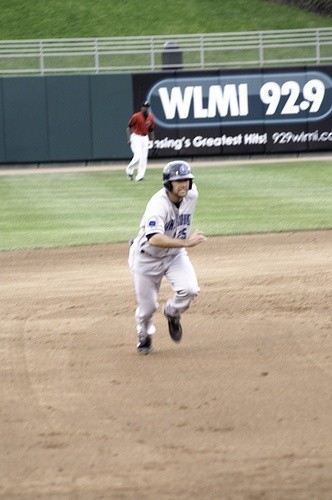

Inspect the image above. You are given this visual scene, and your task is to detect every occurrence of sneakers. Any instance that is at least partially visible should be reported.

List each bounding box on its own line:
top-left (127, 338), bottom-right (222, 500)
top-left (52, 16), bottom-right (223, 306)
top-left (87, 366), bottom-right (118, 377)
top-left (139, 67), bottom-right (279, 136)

top-left (137, 335), bottom-right (151, 352)
top-left (164, 305), bottom-right (182, 341)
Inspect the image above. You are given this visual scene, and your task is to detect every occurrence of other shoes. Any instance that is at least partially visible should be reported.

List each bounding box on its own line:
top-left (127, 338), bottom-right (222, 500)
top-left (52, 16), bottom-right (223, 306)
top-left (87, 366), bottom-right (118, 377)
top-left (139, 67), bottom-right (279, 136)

top-left (135, 177), bottom-right (143, 181)
top-left (127, 175), bottom-right (132, 181)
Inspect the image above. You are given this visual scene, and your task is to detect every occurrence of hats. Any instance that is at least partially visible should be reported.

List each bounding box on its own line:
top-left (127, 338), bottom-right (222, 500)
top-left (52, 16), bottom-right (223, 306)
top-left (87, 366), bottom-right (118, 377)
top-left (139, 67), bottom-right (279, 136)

top-left (140, 101), bottom-right (150, 107)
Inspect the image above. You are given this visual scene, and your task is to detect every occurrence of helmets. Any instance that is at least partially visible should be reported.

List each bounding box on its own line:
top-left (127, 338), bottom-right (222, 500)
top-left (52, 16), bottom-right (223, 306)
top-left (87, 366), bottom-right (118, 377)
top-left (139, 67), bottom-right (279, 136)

top-left (163, 160), bottom-right (194, 190)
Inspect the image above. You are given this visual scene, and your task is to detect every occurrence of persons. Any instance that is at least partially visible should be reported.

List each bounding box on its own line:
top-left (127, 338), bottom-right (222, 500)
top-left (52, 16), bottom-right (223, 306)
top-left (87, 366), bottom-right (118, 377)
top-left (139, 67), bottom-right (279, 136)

top-left (127, 159), bottom-right (208, 356)
top-left (125, 101), bottom-right (155, 182)
